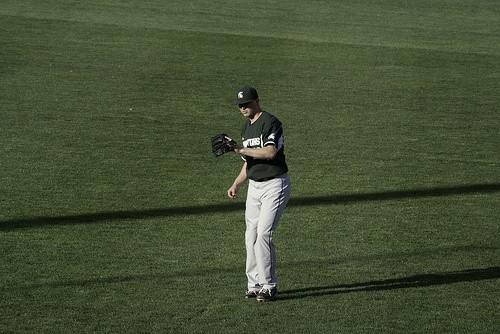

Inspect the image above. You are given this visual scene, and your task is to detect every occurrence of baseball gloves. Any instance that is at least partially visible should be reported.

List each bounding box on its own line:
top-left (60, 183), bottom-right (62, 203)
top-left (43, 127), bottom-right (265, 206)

top-left (209, 133), bottom-right (238, 156)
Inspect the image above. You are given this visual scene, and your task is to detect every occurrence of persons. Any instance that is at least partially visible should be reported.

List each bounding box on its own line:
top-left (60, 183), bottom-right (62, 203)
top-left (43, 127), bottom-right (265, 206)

top-left (211, 87), bottom-right (289, 302)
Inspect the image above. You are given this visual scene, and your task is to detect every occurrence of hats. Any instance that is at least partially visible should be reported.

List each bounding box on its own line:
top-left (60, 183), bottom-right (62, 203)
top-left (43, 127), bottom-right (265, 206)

top-left (233, 87), bottom-right (259, 105)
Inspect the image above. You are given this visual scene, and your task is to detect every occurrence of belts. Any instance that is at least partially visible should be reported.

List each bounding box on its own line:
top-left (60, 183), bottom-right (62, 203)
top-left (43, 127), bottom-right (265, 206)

top-left (254, 175), bottom-right (275, 182)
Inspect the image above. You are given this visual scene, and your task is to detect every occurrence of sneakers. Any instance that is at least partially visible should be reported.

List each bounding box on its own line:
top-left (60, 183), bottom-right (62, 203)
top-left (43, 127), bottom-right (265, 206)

top-left (244, 282), bottom-right (278, 302)
